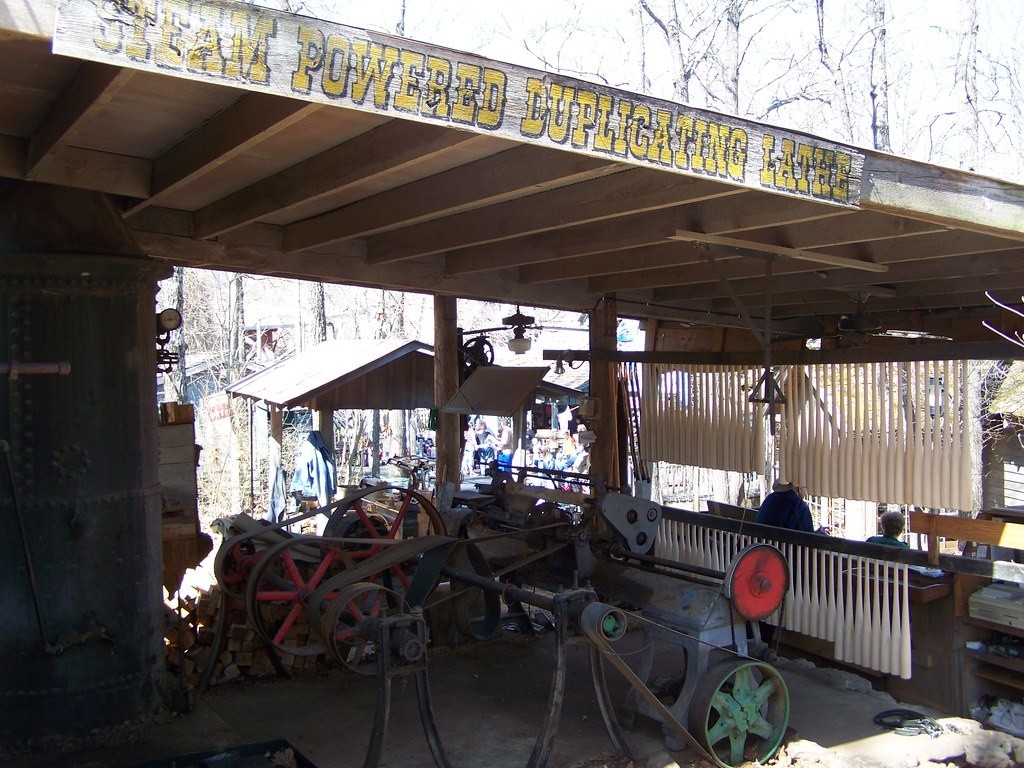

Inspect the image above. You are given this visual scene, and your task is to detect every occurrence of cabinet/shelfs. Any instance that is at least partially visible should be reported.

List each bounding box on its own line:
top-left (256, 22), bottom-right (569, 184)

top-left (840, 567), bottom-right (1024, 741)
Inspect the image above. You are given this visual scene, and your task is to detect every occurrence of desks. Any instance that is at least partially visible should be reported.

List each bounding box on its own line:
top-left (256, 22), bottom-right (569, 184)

top-left (359, 497), bottom-right (419, 539)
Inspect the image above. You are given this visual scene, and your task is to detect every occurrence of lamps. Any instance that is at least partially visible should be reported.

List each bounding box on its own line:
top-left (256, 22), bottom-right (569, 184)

top-left (502, 305), bottom-right (534, 354)
top-left (925, 376), bottom-right (946, 420)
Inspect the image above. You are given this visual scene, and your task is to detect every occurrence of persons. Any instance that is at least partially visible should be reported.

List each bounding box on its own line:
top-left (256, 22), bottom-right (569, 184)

top-left (415, 409), bottom-right (593, 493)
top-left (755, 476), bottom-right (834, 539)
top-left (865, 510), bottom-right (912, 552)
